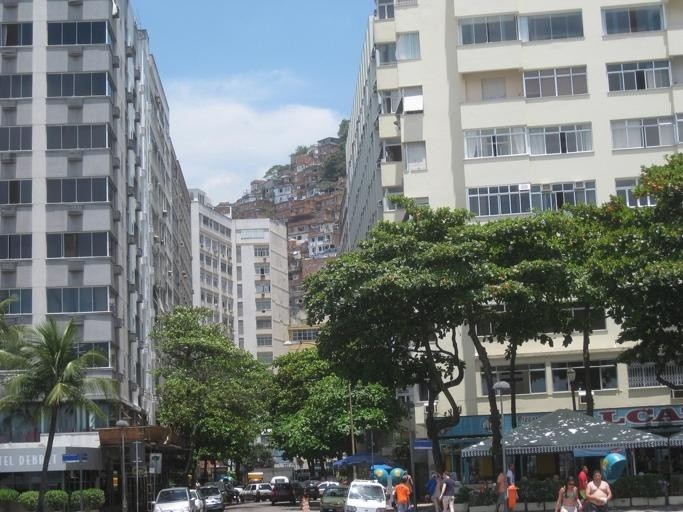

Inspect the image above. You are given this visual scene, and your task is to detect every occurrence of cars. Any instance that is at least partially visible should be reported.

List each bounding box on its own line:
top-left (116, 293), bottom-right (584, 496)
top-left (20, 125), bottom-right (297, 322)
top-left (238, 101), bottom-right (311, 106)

top-left (188, 475), bottom-right (348, 512)
top-left (149, 486), bottom-right (198, 512)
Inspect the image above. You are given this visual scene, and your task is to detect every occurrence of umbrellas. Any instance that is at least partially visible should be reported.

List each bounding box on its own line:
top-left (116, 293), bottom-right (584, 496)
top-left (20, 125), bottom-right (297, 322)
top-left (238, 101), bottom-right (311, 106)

top-left (332, 451), bottom-right (399, 481)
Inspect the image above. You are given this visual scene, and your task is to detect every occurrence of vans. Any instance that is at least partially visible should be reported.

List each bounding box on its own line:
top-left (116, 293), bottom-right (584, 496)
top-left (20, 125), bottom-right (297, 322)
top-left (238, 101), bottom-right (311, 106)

top-left (344, 478), bottom-right (387, 512)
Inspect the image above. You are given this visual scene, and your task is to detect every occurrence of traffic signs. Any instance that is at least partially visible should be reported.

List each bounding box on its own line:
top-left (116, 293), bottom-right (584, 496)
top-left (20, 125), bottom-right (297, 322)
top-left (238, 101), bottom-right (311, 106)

top-left (62, 453), bottom-right (89, 463)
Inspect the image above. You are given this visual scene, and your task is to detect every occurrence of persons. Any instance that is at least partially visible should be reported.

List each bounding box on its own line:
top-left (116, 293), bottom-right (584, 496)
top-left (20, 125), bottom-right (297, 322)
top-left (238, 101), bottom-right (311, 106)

top-left (404, 474), bottom-right (414, 508)
top-left (430, 473), bottom-right (445, 511)
top-left (584, 469), bottom-right (613, 512)
top-left (575, 465), bottom-right (590, 512)
top-left (506, 462), bottom-right (514, 487)
top-left (392, 473), bottom-right (410, 512)
top-left (505, 477), bottom-right (519, 512)
top-left (493, 465), bottom-right (509, 511)
top-left (437, 470), bottom-right (455, 511)
top-left (554, 474), bottom-right (584, 511)
top-left (424, 473), bottom-right (437, 503)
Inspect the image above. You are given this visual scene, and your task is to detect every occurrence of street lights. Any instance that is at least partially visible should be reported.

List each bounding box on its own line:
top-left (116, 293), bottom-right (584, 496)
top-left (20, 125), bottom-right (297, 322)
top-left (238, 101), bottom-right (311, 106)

top-left (492, 378), bottom-right (513, 481)
top-left (281, 338), bottom-right (359, 480)
top-left (115, 419), bottom-right (133, 511)
top-left (565, 366), bottom-right (578, 411)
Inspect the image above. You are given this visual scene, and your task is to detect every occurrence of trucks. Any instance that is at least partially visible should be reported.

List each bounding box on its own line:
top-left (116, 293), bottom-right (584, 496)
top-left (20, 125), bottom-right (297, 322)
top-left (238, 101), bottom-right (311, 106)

top-left (246, 471), bottom-right (264, 483)
